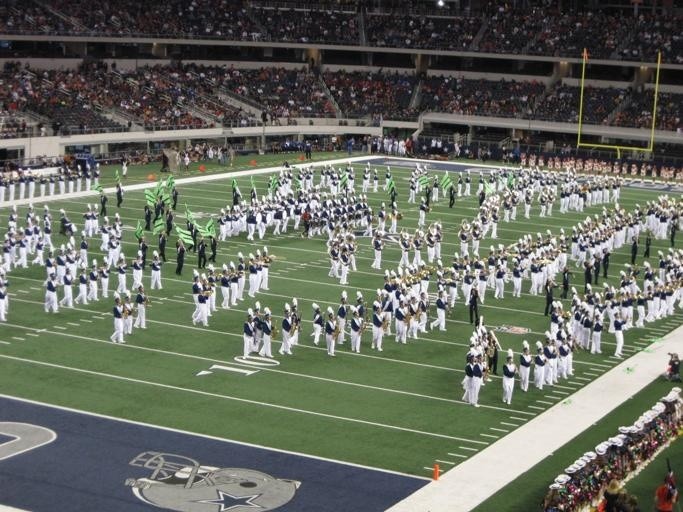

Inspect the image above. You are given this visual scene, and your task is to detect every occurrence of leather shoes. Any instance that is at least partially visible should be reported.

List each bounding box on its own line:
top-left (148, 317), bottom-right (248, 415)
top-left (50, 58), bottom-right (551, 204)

top-left (44, 285), bottom-right (164, 314)
top-left (217, 220), bottom-right (369, 242)
top-left (109, 325), bottom-right (148, 344)
top-left (241, 322), bottom-right (448, 361)
top-left (574, 303), bottom-right (683, 359)
top-left (191, 285), bottom-right (270, 327)
top-left (460, 367), bottom-right (576, 409)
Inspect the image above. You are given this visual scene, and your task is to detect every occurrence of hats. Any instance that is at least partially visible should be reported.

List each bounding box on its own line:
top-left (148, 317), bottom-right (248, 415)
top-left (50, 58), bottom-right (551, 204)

top-left (0, 163), bottom-right (101, 179)
top-left (49, 250), bottom-right (160, 277)
top-left (465, 300), bottom-right (574, 360)
top-left (247, 193), bottom-right (682, 321)
top-left (570, 247), bottom-right (683, 318)
top-left (193, 245), bottom-right (269, 281)
top-left (548, 386), bottom-right (682, 491)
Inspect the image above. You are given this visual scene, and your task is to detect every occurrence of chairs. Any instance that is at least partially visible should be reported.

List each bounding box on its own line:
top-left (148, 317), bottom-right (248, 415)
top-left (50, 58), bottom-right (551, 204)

top-left (2, 1), bottom-right (681, 162)
